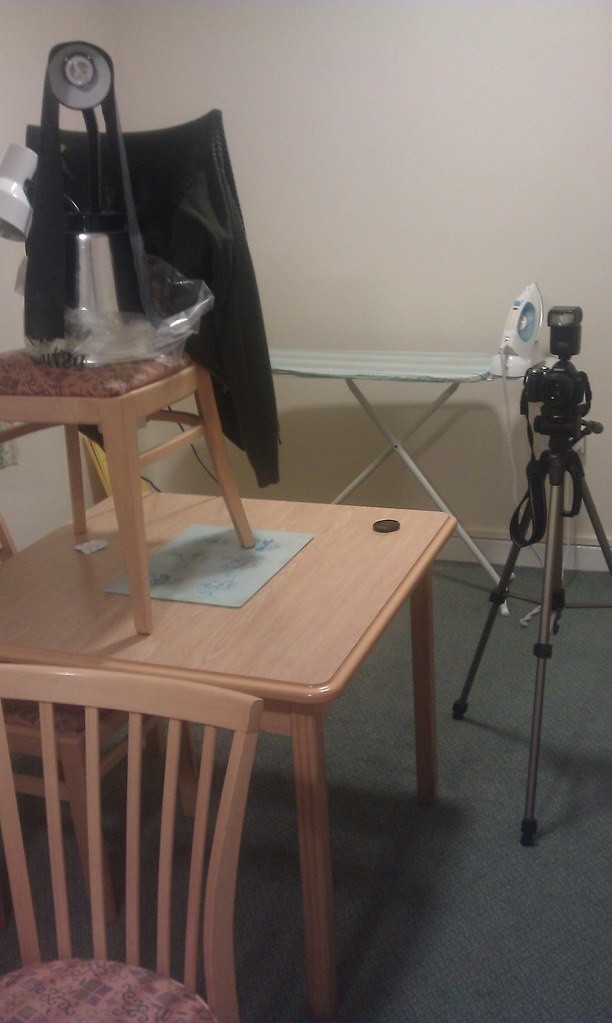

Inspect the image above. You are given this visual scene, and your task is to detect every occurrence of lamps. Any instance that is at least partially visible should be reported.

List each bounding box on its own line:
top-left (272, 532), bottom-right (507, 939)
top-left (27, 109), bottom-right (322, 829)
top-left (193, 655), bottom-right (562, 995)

top-left (39, 40), bottom-right (159, 366)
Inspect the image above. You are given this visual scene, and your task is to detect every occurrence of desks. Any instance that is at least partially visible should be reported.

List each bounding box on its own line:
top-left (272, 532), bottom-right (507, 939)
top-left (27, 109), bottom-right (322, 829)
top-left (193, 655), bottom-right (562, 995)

top-left (0, 491), bottom-right (458, 1023)
top-left (269, 346), bottom-right (512, 619)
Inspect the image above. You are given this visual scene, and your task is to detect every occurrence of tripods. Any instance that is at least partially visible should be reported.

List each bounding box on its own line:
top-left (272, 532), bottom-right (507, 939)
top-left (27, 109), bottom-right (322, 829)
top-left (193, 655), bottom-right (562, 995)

top-left (451, 406), bottom-right (612, 848)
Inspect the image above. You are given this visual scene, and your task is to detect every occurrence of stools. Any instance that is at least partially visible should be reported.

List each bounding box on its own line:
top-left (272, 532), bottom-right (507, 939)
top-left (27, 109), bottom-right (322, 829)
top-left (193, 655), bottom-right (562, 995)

top-left (0, 348), bottom-right (256, 635)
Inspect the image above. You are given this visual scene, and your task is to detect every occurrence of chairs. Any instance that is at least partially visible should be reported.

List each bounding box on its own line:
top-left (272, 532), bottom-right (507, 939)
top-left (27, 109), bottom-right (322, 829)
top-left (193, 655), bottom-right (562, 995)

top-left (0, 663), bottom-right (263, 1023)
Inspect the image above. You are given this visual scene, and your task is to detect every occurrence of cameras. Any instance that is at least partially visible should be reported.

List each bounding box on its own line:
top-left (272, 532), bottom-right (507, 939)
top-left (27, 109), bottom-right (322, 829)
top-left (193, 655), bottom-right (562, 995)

top-left (524, 305), bottom-right (585, 407)
